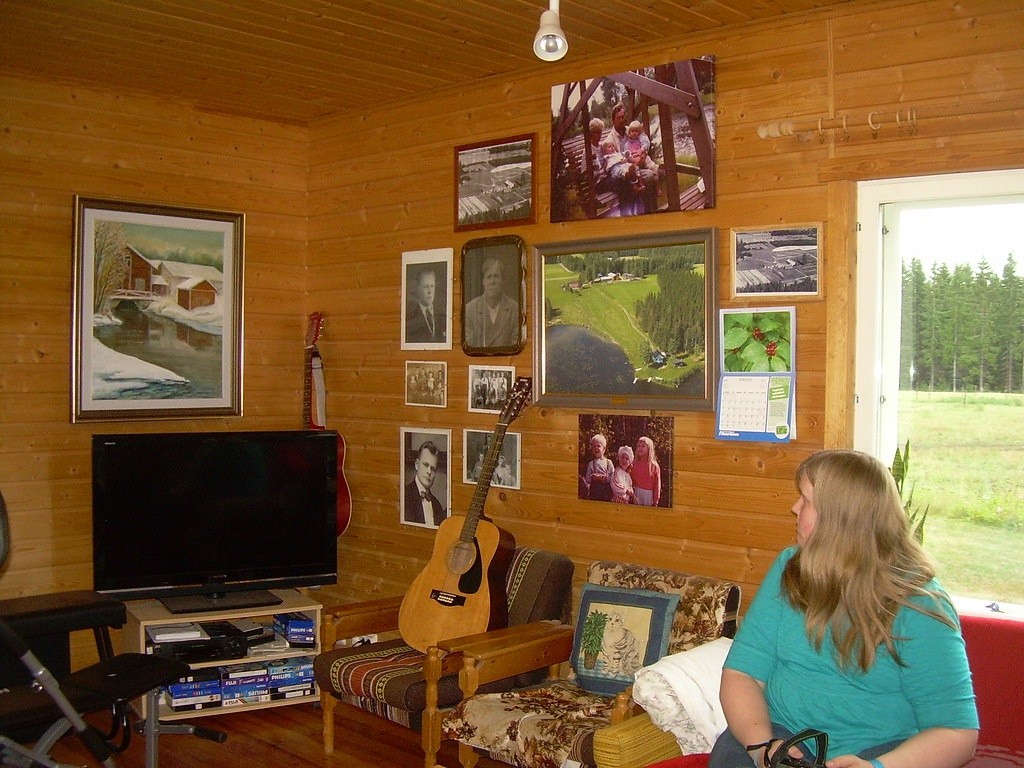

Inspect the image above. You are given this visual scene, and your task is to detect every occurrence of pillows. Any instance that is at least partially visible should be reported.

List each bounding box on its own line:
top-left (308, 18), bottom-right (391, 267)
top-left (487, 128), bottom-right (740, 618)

top-left (632, 636), bottom-right (733, 757)
top-left (569, 582), bottom-right (682, 697)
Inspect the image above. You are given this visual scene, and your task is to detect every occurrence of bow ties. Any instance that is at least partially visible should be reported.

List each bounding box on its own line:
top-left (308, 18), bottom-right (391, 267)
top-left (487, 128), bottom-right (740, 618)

top-left (420, 491), bottom-right (433, 501)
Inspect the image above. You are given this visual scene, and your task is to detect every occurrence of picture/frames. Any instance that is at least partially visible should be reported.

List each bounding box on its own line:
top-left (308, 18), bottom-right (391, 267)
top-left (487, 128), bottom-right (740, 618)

top-left (452, 132), bottom-right (539, 233)
top-left (531, 226), bottom-right (719, 413)
top-left (459, 234), bottom-right (527, 357)
top-left (729, 220), bottom-right (824, 303)
top-left (67, 192), bottom-right (247, 425)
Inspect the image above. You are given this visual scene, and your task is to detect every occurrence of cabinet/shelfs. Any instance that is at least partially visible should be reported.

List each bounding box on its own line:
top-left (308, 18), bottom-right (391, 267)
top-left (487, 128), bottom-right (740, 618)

top-left (121, 588), bottom-right (323, 736)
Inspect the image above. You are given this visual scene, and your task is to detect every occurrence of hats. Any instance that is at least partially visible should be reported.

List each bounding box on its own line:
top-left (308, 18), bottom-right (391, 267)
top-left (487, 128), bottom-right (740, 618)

top-left (618, 446), bottom-right (634, 463)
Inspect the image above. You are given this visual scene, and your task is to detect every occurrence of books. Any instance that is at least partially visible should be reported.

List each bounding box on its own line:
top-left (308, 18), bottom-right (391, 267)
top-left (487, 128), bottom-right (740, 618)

top-left (145, 611), bottom-right (315, 713)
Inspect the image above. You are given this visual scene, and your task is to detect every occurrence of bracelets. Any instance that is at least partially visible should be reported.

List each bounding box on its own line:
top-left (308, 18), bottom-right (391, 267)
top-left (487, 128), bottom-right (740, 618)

top-left (754, 741), bottom-right (769, 768)
top-left (869, 757), bottom-right (885, 768)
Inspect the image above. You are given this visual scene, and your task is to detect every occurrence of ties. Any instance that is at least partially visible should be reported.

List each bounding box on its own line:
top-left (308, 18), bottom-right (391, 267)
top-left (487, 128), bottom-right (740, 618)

top-left (425, 306), bottom-right (433, 332)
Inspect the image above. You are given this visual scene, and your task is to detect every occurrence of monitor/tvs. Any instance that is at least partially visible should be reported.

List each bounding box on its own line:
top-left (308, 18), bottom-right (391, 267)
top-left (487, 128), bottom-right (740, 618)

top-left (92, 429), bottom-right (340, 612)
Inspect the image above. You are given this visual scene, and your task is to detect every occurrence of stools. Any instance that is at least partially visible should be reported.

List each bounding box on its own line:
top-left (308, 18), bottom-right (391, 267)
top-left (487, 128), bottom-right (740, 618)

top-left (0, 590), bottom-right (131, 753)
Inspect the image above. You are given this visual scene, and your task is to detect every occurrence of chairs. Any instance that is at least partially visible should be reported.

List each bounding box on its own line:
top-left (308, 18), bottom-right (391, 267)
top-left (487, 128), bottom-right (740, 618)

top-left (441, 560), bottom-right (742, 768)
top-left (312, 542), bottom-right (574, 768)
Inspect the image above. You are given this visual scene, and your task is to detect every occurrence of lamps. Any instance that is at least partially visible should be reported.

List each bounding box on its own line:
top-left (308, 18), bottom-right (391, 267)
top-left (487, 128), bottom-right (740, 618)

top-left (532, 0), bottom-right (568, 62)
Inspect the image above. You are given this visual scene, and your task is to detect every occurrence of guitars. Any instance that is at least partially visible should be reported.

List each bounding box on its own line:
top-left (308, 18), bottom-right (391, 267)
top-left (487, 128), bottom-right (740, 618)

top-left (400, 376), bottom-right (534, 652)
top-left (305, 311), bottom-right (351, 536)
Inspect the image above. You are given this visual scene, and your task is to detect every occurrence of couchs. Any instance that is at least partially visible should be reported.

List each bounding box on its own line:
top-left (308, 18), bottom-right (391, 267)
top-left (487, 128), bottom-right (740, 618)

top-left (593, 614), bottom-right (1024, 768)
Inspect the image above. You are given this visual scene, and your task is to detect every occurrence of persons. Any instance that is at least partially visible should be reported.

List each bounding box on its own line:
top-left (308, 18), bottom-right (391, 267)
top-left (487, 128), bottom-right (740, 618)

top-left (489, 454), bottom-right (516, 486)
top-left (471, 371), bottom-right (510, 409)
top-left (406, 269), bottom-right (446, 343)
top-left (584, 434), bottom-right (661, 507)
top-left (471, 453), bottom-right (484, 482)
top-left (465, 257), bottom-right (518, 346)
top-left (707, 448), bottom-right (980, 768)
top-left (407, 364), bottom-right (444, 405)
top-left (589, 102), bottom-right (663, 216)
top-left (405, 440), bottom-right (446, 526)
